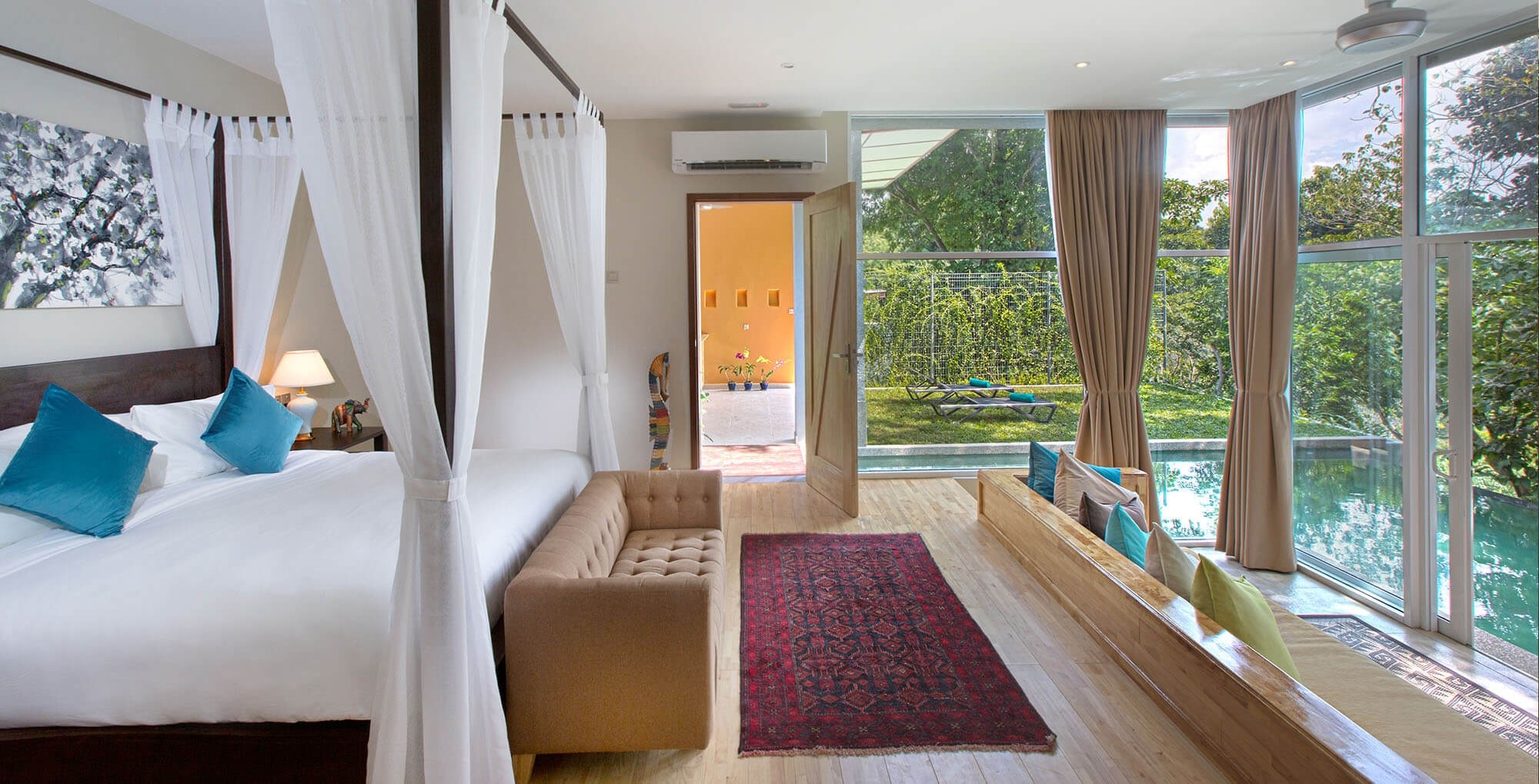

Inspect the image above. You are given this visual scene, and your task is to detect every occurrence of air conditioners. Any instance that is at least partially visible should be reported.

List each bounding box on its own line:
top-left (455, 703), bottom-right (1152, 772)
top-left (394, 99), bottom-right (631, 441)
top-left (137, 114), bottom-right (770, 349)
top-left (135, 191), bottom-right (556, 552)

top-left (671, 129), bottom-right (827, 175)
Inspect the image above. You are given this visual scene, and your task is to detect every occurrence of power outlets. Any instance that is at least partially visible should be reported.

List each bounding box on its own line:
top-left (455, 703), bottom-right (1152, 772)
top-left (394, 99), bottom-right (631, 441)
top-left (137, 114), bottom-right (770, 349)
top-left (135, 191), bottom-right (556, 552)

top-left (275, 395), bottom-right (281, 403)
top-left (744, 324), bottom-right (749, 330)
top-left (281, 393), bottom-right (291, 408)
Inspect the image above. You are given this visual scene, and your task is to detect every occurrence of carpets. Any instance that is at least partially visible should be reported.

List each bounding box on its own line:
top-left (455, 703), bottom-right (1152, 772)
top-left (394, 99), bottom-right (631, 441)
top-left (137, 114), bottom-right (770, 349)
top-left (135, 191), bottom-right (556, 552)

top-left (1297, 615), bottom-right (1539, 760)
top-left (736, 532), bottom-right (1059, 757)
top-left (701, 444), bottom-right (806, 477)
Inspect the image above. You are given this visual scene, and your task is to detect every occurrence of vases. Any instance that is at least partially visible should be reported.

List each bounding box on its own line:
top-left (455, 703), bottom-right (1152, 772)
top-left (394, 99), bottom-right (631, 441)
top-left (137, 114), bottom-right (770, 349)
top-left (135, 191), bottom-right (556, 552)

top-left (760, 382), bottom-right (769, 390)
top-left (744, 381), bottom-right (753, 390)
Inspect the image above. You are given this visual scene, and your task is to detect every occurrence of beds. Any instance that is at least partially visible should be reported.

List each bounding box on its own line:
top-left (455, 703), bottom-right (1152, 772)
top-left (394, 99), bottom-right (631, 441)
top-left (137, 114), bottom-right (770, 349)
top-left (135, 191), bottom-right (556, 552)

top-left (0, 342), bottom-right (617, 784)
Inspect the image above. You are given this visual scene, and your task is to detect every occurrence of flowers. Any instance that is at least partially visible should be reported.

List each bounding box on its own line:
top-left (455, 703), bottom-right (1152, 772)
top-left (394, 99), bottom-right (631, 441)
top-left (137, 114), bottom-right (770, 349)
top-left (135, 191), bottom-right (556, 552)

top-left (733, 346), bottom-right (785, 382)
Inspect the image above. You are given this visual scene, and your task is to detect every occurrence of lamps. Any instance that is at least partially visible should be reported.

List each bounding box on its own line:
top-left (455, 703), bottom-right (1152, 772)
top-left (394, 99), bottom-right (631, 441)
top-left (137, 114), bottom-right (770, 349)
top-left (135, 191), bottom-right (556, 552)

top-left (268, 349), bottom-right (335, 440)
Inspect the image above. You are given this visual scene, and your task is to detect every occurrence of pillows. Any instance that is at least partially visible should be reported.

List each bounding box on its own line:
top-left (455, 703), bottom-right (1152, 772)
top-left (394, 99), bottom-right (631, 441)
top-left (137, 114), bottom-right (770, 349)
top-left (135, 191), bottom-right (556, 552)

top-left (1026, 437), bottom-right (1121, 506)
top-left (199, 366), bottom-right (304, 476)
top-left (1104, 501), bottom-right (1153, 570)
top-left (1145, 520), bottom-right (1198, 602)
top-left (968, 377), bottom-right (1036, 403)
top-left (130, 385), bottom-right (275, 489)
top-left (0, 383), bottom-right (158, 540)
top-left (1079, 490), bottom-right (1148, 542)
top-left (0, 423), bottom-right (62, 549)
top-left (1052, 447), bottom-right (1144, 524)
top-left (1191, 553), bottom-right (1303, 685)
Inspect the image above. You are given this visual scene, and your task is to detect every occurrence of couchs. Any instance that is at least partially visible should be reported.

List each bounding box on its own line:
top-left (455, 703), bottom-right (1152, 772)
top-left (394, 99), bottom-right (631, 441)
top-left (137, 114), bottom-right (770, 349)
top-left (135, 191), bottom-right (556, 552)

top-left (977, 437), bottom-right (1539, 784)
top-left (503, 469), bottom-right (723, 755)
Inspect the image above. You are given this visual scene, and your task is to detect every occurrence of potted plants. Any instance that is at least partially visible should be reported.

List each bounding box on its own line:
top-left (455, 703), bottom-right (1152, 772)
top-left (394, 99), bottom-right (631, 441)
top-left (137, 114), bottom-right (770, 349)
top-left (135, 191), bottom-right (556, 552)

top-left (717, 364), bottom-right (737, 390)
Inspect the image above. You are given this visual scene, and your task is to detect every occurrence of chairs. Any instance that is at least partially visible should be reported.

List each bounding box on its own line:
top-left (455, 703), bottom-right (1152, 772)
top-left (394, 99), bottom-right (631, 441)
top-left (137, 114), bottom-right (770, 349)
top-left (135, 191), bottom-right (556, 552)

top-left (926, 379), bottom-right (1057, 425)
top-left (904, 365), bottom-right (1015, 406)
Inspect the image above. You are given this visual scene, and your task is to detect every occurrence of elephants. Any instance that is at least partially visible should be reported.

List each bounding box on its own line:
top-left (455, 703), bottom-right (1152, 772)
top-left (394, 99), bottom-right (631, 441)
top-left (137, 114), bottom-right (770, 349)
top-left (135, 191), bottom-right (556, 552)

top-left (331, 398), bottom-right (370, 433)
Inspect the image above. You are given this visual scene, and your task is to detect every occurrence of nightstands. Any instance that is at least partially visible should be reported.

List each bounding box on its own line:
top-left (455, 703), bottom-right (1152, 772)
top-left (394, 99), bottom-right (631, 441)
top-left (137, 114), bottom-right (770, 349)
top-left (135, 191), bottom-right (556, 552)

top-left (290, 426), bottom-right (389, 452)
